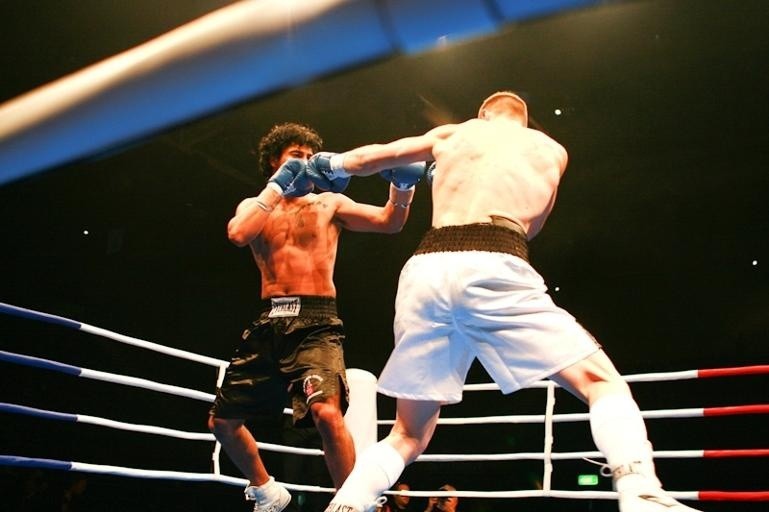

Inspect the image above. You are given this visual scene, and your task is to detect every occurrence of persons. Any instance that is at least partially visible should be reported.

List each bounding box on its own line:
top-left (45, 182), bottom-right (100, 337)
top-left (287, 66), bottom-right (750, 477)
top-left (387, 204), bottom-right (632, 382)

top-left (376, 482), bottom-right (460, 512)
top-left (305, 91), bottom-right (701, 512)
top-left (209, 123), bottom-right (426, 512)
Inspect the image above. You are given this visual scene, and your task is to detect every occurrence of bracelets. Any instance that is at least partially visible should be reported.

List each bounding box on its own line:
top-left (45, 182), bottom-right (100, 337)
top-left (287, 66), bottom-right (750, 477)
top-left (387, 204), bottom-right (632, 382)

top-left (255, 199), bottom-right (272, 213)
top-left (388, 200), bottom-right (410, 209)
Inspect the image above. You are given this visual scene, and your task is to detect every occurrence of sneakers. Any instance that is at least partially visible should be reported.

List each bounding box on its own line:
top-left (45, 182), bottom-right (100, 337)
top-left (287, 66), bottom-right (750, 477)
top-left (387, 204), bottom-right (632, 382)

top-left (246, 476), bottom-right (292, 512)
top-left (621, 489), bottom-right (702, 512)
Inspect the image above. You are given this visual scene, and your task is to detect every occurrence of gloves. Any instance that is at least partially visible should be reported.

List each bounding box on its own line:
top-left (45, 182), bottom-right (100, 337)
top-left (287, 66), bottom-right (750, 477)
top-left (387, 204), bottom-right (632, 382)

top-left (380, 161), bottom-right (426, 206)
top-left (306, 151), bottom-right (352, 193)
top-left (255, 158), bottom-right (314, 212)
top-left (426, 160), bottom-right (436, 185)
